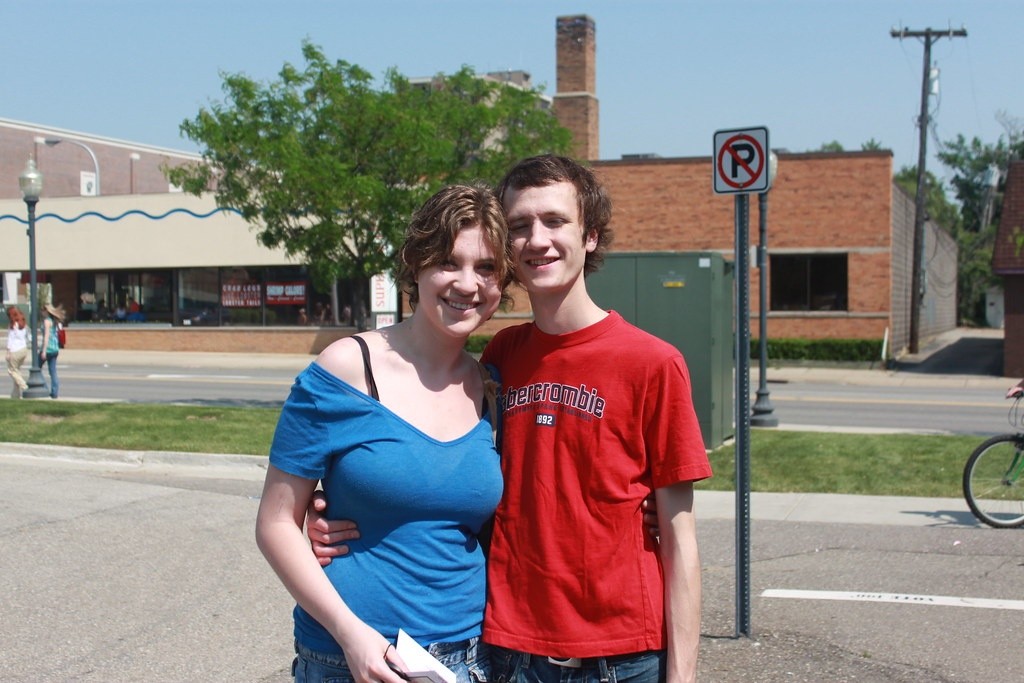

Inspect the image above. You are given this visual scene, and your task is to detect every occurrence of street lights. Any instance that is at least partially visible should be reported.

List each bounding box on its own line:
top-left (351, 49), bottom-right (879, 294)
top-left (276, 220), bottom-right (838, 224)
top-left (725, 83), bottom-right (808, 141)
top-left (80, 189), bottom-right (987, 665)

top-left (749, 152), bottom-right (779, 427)
top-left (35, 136), bottom-right (101, 196)
top-left (19, 158), bottom-right (50, 398)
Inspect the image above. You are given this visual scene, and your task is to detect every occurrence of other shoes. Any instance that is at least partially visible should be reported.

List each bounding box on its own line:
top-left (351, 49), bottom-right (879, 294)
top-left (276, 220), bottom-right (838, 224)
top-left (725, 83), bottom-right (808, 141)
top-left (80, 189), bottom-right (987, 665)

top-left (49, 393), bottom-right (58, 398)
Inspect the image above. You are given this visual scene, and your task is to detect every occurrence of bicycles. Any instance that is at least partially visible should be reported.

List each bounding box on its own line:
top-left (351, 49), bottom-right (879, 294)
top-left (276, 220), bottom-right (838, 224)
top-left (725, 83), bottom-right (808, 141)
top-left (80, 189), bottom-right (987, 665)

top-left (962, 391), bottom-right (1024, 527)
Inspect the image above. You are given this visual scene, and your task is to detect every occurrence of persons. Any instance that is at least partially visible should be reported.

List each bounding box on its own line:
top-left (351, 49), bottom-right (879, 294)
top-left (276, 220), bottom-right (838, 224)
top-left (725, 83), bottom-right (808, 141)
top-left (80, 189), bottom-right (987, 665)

top-left (306, 153), bottom-right (713, 683)
top-left (189, 307), bottom-right (215, 325)
top-left (96, 297), bottom-right (139, 323)
top-left (38, 302), bottom-right (66, 399)
top-left (255, 185), bottom-right (659, 683)
top-left (297, 302), bottom-right (351, 325)
top-left (1006, 378), bottom-right (1024, 398)
top-left (5, 305), bottom-right (32, 398)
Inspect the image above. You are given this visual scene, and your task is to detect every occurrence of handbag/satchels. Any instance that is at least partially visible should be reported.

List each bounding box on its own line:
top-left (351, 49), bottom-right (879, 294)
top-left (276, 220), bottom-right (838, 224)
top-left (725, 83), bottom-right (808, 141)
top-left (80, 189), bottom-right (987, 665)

top-left (54, 317), bottom-right (66, 349)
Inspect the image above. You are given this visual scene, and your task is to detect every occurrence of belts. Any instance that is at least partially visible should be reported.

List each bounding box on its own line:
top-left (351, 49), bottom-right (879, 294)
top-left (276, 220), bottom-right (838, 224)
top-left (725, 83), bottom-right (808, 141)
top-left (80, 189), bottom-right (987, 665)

top-left (541, 656), bottom-right (597, 670)
